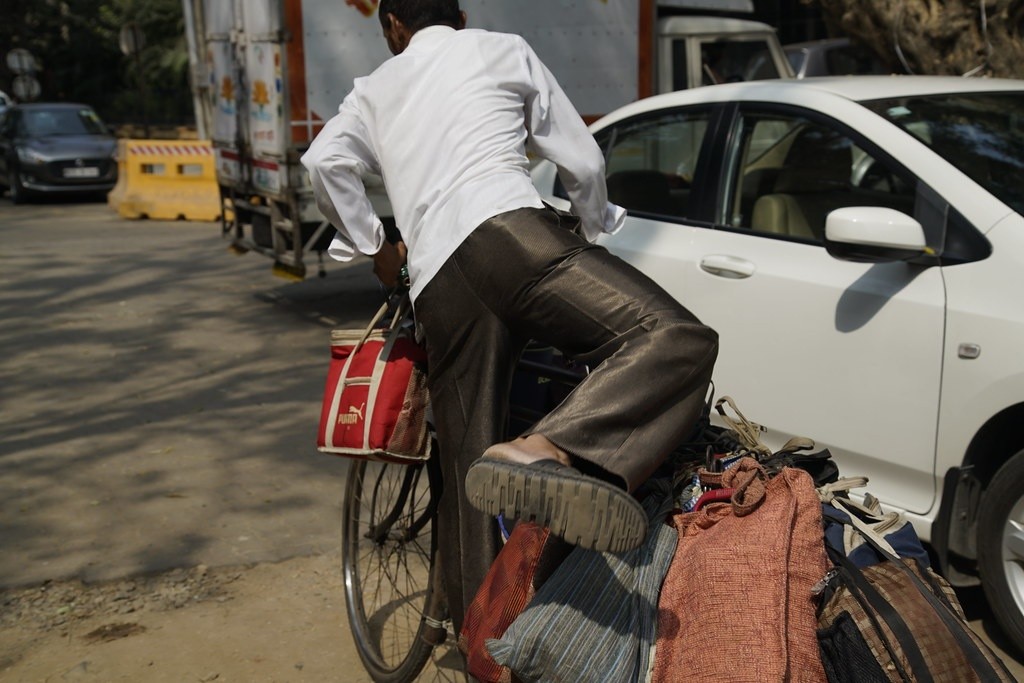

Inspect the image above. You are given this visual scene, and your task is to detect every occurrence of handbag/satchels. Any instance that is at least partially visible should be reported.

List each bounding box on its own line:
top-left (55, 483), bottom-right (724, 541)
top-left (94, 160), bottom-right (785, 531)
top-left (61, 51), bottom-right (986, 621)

top-left (483, 515), bottom-right (678, 683)
top-left (317, 298), bottom-right (433, 465)
top-left (652, 463), bottom-right (828, 683)
top-left (461, 518), bottom-right (550, 683)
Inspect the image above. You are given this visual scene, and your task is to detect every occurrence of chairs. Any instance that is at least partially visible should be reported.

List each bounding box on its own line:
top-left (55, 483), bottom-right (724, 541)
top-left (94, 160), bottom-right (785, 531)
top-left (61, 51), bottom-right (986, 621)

top-left (751, 124), bottom-right (876, 244)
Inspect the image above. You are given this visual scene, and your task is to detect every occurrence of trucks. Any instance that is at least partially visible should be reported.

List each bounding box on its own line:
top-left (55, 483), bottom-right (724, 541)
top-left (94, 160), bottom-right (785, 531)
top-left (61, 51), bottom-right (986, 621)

top-left (182, 1), bottom-right (799, 278)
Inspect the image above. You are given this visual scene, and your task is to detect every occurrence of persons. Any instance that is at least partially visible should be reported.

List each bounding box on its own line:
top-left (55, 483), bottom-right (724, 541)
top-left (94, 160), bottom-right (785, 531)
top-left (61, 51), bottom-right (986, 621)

top-left (296, 0), bottom-right (722, 664)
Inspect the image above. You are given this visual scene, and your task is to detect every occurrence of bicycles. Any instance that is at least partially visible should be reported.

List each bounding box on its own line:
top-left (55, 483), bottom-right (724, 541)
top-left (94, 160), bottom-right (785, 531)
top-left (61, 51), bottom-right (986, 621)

top-left (339, 205), bottom-right (737, 683)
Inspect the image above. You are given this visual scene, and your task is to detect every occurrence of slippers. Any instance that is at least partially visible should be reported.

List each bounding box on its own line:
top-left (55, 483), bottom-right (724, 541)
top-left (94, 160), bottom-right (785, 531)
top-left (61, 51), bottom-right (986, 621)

top-left (468, 457), bottom-right (647, 554)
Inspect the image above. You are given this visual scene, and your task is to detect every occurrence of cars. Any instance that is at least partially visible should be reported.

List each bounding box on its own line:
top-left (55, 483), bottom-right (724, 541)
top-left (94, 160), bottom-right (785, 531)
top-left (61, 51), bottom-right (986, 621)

top-left (0, 102), bottom-right (119, 203)
top-left (531, 70), bottom-right (1022, 663)
